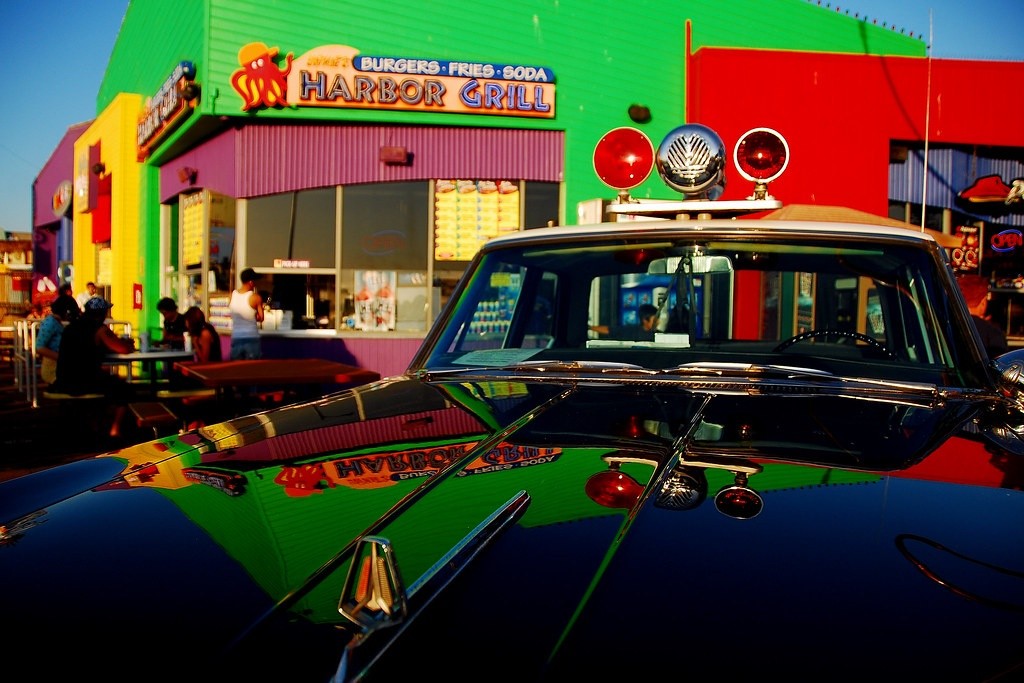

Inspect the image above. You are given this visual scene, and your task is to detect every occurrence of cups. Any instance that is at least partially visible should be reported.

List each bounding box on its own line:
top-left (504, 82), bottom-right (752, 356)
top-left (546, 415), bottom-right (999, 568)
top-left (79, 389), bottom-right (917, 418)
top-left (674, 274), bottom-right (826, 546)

top-left (184, 335), bottom-right (192, 353)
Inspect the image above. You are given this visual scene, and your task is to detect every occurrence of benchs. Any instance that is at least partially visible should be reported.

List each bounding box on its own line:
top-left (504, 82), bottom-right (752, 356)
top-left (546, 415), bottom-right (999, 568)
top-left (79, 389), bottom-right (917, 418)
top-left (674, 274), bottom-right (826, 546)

top-left (39, 378), bottom-right (297, 440)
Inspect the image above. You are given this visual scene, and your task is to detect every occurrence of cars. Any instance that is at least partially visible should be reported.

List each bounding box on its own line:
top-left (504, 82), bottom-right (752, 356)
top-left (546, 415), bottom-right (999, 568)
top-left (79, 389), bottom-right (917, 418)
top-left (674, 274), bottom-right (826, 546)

top-left (0, 122), bottom-right (1024, 683)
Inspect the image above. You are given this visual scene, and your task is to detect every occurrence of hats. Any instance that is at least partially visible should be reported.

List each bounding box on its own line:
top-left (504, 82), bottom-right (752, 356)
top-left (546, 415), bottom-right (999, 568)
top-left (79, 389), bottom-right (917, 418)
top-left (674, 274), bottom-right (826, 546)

top-left (84, 297), bottom-right (114, 312)
top-left (241, 268), bottom-right (262, 284)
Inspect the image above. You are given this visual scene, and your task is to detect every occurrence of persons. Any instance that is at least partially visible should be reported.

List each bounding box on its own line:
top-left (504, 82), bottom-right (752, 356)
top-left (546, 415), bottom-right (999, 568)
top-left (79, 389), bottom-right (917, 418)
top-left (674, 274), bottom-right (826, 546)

top-left (228, 268), bottom-right (264, 360)
top-left (148, 297), bottom-right (222, 403)
top-left (949, 275), bottom-right (1007, 389)
top-left (36, 283), bottom-right (135, 449)
top-left (589, 303), bottom-right (661, 342)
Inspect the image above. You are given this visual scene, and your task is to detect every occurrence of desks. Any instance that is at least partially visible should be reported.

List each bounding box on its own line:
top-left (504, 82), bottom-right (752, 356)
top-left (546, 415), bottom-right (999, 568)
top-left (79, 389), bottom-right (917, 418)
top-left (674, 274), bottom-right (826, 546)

top-left (101, 348), bottom-right (193, 404)
top-left (171, 357), bottom-right (381, 425)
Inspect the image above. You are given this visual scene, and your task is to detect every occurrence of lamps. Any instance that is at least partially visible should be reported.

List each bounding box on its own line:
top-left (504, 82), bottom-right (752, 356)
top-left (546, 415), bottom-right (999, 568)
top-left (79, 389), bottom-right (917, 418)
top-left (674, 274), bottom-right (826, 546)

top-left (90, 163), bottom-right (106, 174)
top-left (379, 146), bottom-right (407, 163)
top-left (176, 166), bottom-right (198, 183)
